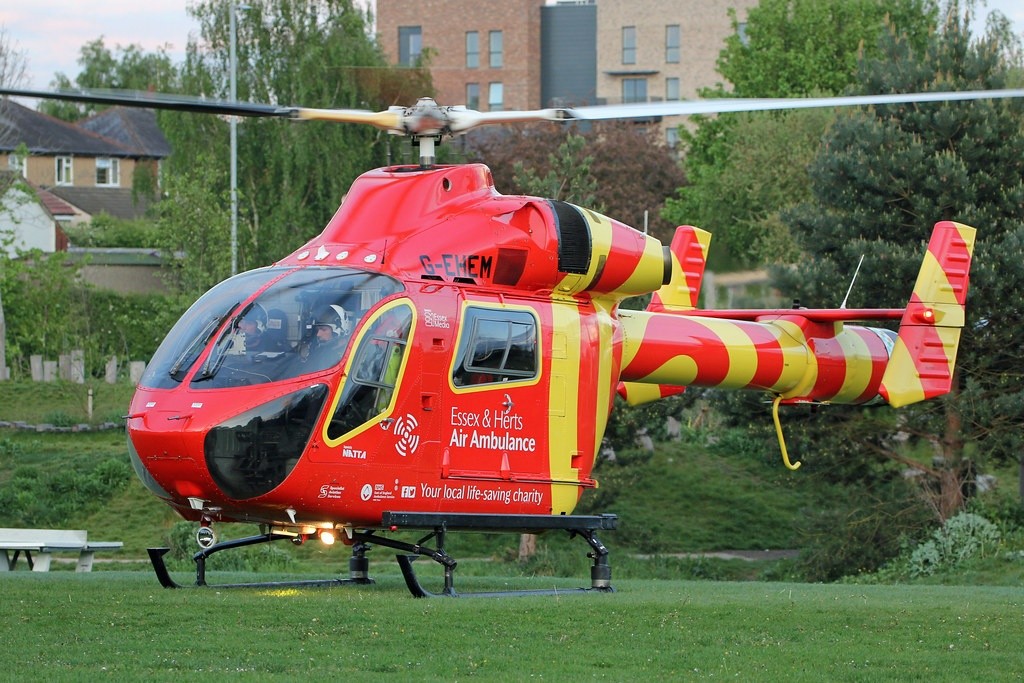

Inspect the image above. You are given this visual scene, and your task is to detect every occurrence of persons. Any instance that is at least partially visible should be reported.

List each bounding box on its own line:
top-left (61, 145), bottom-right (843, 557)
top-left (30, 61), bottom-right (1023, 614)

top-left (207, 303), bottom-right (410, 476)
top-left (467, 340), bottom-right (495, 385)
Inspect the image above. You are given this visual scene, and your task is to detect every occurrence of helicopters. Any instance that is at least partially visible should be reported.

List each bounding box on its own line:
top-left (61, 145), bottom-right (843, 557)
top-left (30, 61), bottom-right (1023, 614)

top-left (0, 81), bottom-right (1024, 604)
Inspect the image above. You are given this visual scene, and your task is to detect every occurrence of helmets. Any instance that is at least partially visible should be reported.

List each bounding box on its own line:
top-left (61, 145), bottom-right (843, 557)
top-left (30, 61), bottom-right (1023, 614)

top-left (241, 302), bottom-right (267, 336)
top-left (310, 305), bottom-right (351, 338)
top-left (370, 317), bottom-right (402, 352)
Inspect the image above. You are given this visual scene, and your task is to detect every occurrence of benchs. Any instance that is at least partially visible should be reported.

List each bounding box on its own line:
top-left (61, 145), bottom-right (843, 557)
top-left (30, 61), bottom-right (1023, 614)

top-left (0, 527), bottom-right (123, 571)
